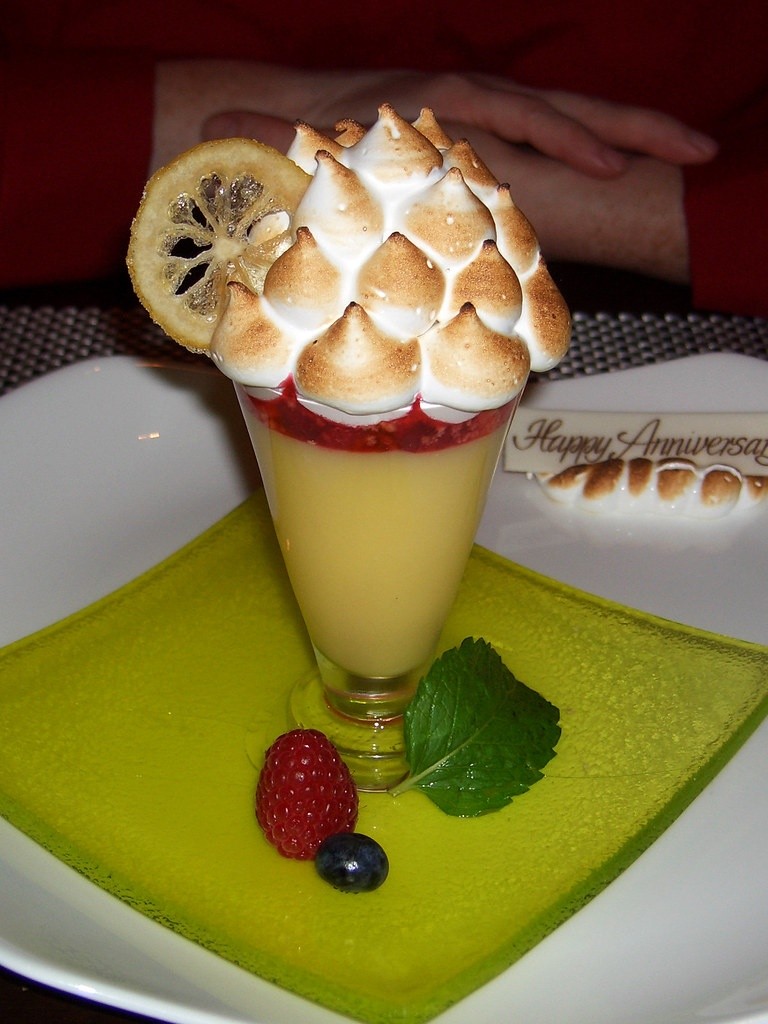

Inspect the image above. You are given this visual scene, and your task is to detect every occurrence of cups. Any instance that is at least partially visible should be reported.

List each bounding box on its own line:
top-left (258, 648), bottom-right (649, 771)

top-left (230, 376), bottom-right (526, 799)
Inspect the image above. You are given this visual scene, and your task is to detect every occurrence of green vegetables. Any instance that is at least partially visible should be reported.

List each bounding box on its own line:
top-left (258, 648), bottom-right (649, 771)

top-left (386, 636), bottom-right (562, 818)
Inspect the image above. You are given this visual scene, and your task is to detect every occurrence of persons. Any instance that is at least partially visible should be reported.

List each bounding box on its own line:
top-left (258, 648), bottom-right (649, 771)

top-left (0, 0), bottom-right (768, 315)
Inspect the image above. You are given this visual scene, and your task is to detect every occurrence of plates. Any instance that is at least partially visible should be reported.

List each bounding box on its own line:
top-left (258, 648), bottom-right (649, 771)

top-left (0, 352), bottom-right (768, 1024)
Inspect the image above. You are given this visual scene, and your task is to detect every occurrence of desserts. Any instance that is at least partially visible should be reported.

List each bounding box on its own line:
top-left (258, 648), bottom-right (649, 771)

top-left (206, 99), bottom-right (571, 425)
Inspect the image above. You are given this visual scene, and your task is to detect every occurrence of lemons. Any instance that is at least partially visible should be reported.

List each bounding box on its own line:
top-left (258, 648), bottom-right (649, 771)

top-left (129, 136), bottom-right (312, 355)
